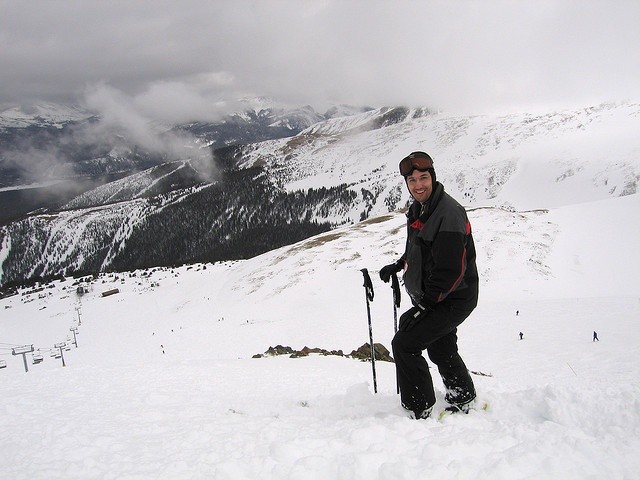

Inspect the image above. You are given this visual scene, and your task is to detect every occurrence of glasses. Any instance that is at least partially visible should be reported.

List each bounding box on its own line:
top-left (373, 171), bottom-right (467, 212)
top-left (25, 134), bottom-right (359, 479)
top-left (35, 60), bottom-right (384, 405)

top-left (399, 157), bottom-right (431, 176)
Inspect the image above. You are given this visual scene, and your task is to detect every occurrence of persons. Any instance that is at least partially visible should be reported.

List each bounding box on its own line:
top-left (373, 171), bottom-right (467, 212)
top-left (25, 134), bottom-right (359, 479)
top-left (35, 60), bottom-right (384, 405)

top-left (379, 152), bottom-right (480, 419)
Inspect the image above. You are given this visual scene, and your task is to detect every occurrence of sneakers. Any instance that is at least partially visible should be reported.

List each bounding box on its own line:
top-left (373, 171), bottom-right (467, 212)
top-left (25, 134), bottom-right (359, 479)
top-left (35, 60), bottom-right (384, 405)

top-left (445, 404), bottom-right (469, 415)
top-left (414, 406), bottom-right (433, 420)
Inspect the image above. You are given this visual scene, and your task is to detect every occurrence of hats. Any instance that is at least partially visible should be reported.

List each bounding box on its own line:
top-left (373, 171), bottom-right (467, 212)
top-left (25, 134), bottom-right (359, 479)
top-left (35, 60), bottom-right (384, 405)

top-left (404, 151), bottom-right (436, 203)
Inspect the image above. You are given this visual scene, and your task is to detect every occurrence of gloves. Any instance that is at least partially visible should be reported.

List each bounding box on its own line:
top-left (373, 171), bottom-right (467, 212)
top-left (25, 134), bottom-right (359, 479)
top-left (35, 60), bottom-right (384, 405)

top-left (379, 259), bottom-right (404, 283)
top-left (399, 300), bottom-right (431, 332)
